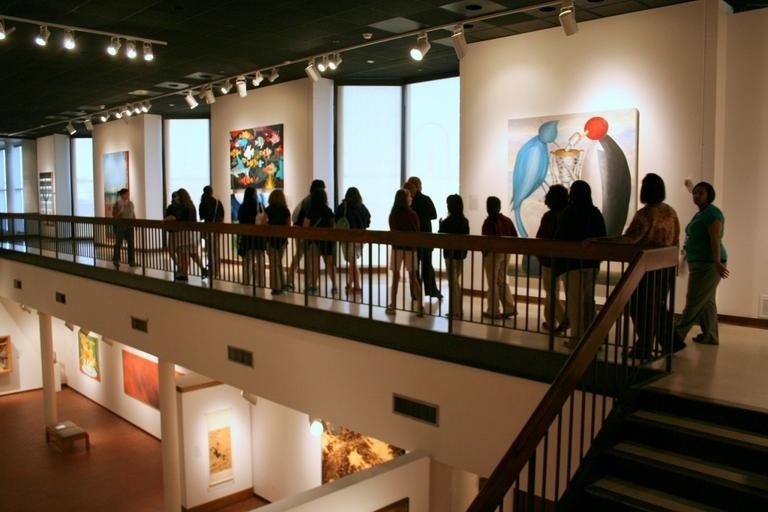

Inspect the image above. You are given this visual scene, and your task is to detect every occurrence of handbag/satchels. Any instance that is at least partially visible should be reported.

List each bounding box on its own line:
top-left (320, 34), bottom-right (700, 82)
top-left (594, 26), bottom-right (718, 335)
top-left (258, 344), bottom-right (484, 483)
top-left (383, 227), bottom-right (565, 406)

top-left (255, 211), bottom-right (269, 225)
top-left (336, 218), bottom-right (350, 230)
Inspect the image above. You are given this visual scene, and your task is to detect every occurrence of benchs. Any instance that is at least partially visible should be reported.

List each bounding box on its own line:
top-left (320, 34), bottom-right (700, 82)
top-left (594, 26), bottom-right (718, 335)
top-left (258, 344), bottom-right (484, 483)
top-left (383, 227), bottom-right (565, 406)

top-left (45, 418), bottom-right (90, 456)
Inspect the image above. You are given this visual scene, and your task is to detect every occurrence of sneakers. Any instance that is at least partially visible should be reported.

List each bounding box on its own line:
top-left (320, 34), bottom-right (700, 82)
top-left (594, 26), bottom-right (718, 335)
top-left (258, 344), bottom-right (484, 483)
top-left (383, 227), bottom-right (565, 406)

top-left (621, 350), bottom-right (653, 359)
top-left (385, 307), bottom-right (396, 315)
top-left (112, 258), bottom-right (119, 270)
top-left (273, 283), bottom-right (360, 297)
top-left (693, 334), bottom-right (704, 343)
top-left (129, 263), bottom-right (138, 267)
top-left (176, 274), bottom-right (187, 280)
top-left (201, 267), bottom-right (208, 279)
top-left (446, 312), bottom-right (461, 318)
top-left (416, 307), bottom-right (424, 316)
top-left (483, 310), bottom-right (516, 319)
top-left (542, 319), bottom-right (580, 349)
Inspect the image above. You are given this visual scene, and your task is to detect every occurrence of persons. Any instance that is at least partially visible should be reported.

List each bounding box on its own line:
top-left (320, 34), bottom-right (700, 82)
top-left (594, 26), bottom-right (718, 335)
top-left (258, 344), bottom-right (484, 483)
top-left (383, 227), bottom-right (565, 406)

top-left (672, 182), bottom-right (731, 345)
top-left (584, 173), bottom-right (686, 357)
top-left (384, 176), bottom-right (606, 349)
top-left (162, 180), bottom-right (371, 297)
top-left (112, 188), bottom-right (140, 267)
